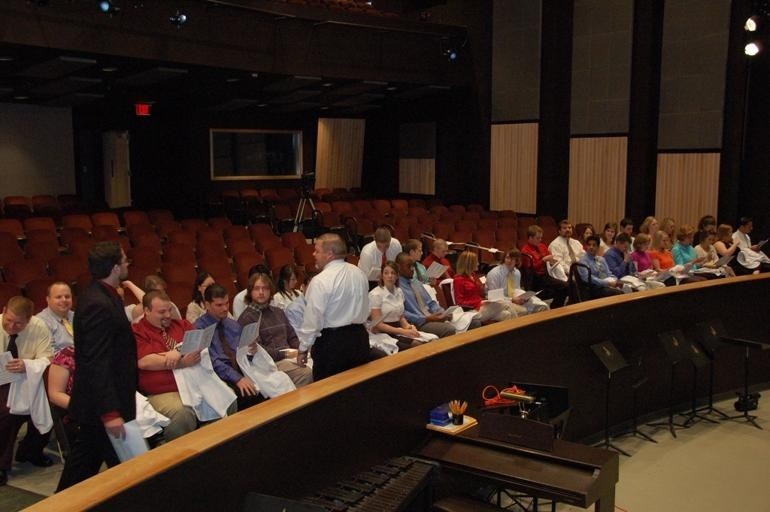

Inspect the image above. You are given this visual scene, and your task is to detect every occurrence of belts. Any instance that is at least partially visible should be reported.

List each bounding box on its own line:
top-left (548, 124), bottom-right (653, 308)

top-left (326, 323), bottom-right (363, 332)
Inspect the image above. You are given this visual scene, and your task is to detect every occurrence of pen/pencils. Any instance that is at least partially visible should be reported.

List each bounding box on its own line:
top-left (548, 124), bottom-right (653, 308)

top-left (448, 400), bottom-right (468, 416)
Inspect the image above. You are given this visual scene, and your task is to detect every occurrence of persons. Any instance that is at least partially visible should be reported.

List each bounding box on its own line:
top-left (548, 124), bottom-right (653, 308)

top-left (185, 227), bottom-right (549, 410)
top-left (1, 240), bottom-right (237, 483)
top-left (519, 214), bottom-right (770, 308)
top-left (54, 242), bottom-right (138, 493)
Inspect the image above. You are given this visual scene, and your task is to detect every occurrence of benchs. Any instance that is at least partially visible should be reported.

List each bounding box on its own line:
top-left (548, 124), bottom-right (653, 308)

top-left (432, 494), bottom-right (504, 512)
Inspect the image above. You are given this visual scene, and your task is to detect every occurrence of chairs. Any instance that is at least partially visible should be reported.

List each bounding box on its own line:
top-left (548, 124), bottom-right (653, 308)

top-left (0, 182), bottom-right (595, 316)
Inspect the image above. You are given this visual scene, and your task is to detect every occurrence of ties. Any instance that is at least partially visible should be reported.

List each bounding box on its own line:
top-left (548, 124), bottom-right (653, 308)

top-left (382, 251), bottom-right (387, 273)
top-left (162, 326), bottom-right (177, 350)
top-left (7, 334), bottom-right (18, 359)
top-left (219, 321), bottom-right (239, 369)
top-left (409, 279), bottom-right (431, 316)
top-left (508, 271), bottom-right (514, 298)
top-left (61, 317), bottom-right (74, 338)
top-left (620, 252), bottom-right (630, 275)
top-left (593, 257), bottom-right (607, 279)
top-left (567, 239), bottom-right (576, 263)
top-left (414, 263), bottom-right (424, 283)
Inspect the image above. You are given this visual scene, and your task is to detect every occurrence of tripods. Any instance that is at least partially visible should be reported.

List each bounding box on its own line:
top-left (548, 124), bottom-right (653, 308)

top-left (294, 192), bottom-right (318, 225)
top-left (605, 343), bottom-right (762, 457)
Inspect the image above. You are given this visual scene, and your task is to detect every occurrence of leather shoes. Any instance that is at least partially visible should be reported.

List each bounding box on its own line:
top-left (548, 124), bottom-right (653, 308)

top-left (15, 445), bottom-right (60, 467)
